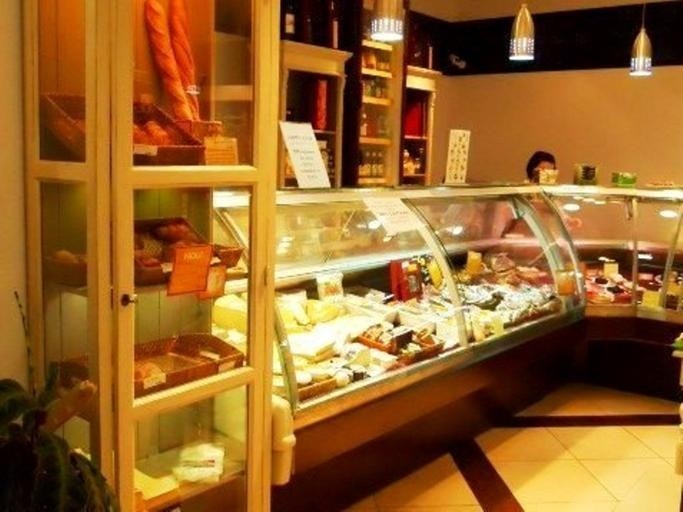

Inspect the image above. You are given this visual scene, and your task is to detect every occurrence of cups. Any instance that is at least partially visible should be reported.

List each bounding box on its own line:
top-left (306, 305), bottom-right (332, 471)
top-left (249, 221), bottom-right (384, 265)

top-left (603, 258), bottom-right (619, 278)
top-left (466, 250), bottom-right (483, 274)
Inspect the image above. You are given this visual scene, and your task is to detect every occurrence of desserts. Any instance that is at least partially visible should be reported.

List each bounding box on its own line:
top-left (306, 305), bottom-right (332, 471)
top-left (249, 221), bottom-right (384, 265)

top-left (212, 247), bottom-right (563, 396)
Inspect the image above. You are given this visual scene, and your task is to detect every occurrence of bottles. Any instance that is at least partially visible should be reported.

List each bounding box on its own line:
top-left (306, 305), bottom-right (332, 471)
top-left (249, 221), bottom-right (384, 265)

top-left (357, 49), bottom-right (392, 178)
top-left (413, 147), bottom-right (425, 174)
top-left (281, 0), bottom-right (301, 42)
top-left (325, 0), bottom-right (342, 49)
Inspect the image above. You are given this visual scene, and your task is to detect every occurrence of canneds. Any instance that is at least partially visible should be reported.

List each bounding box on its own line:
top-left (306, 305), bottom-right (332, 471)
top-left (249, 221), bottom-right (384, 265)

top-left (603, 260), bottom-right (618, 275)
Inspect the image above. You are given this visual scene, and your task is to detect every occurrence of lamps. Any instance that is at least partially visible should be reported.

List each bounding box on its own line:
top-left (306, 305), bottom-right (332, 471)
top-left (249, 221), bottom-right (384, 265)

top-left (507, 0), bottom-right (536, 61)
top-left (629, 0), bottom-right (652, 76)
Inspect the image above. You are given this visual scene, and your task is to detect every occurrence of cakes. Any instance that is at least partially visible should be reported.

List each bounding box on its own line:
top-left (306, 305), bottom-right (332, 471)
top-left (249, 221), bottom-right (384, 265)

top-left (580, 274), bottom-right (682, 320)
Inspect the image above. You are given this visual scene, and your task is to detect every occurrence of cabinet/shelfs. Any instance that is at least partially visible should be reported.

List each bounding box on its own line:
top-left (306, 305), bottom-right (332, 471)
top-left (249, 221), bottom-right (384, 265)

top-left (21, 0), bottom-right (282, 512)
top-left (277, 0), bottom-right (441, 189)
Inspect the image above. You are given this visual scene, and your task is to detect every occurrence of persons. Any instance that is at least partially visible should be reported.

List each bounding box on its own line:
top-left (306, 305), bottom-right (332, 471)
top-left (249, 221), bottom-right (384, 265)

top-left (487, 149), bottom-right (583, 260)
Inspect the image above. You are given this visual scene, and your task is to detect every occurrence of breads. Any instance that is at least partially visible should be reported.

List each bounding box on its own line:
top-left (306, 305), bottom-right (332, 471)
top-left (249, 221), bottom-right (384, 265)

top-left (173, 440), bottom-right (226, 484)
top-left (134, 219), bottom-right (201, 271)
top-left (134, 359), bottom-right (163, 380)
top-left (131, 120), bottom-right (170, 146)
top-left (46, 247), bottom-right (86, 267)
